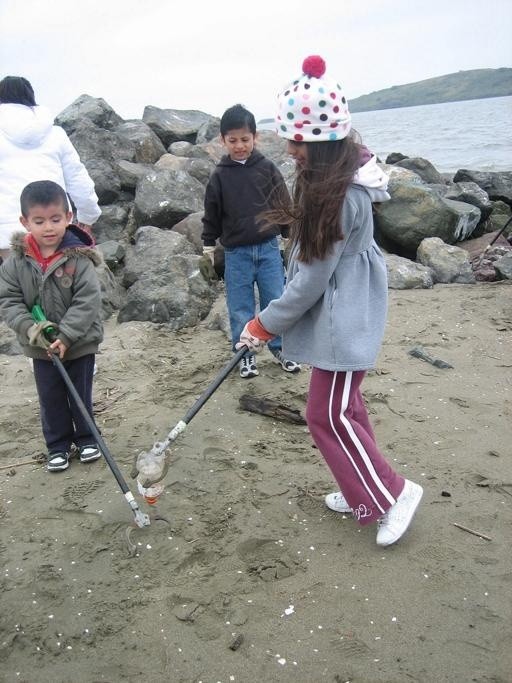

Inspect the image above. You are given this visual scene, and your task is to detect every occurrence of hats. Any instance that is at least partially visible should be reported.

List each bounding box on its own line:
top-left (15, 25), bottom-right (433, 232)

top-left (274, 56), bottom-right (352, 143)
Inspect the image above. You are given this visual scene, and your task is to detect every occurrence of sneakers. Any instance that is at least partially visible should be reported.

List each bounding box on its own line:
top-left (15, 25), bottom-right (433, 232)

top-left (48, 442), bottom-right (78, 472)
top-left (376, 478), bottom-right (424, 547)
top-left (325, 490), bottom-right (353, 512)
top-left (238, 355), bottom-right (259, 378)
top-left (269, 347), bottom-right (302, 372)
top-left (77, 443), bottom-right (102, 463)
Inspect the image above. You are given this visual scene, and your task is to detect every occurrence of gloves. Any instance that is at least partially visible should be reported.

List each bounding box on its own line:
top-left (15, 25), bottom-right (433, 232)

top-left (27, 321), bottom-right (60, 354)
top-left (203, 246), bottom-right (216, 266)
top-left (235, 317), bottom-right (274, 357)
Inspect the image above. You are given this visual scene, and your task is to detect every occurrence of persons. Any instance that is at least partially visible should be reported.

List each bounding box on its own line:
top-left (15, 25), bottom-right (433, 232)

top-left (1, 73), bottom-right (102, 262)
top-left (199, 103), bottom-right (303, 379)
top-left (234, 51), bottom-right (425, 549)
top-left (1, 179), bottom-right (104, 475)
